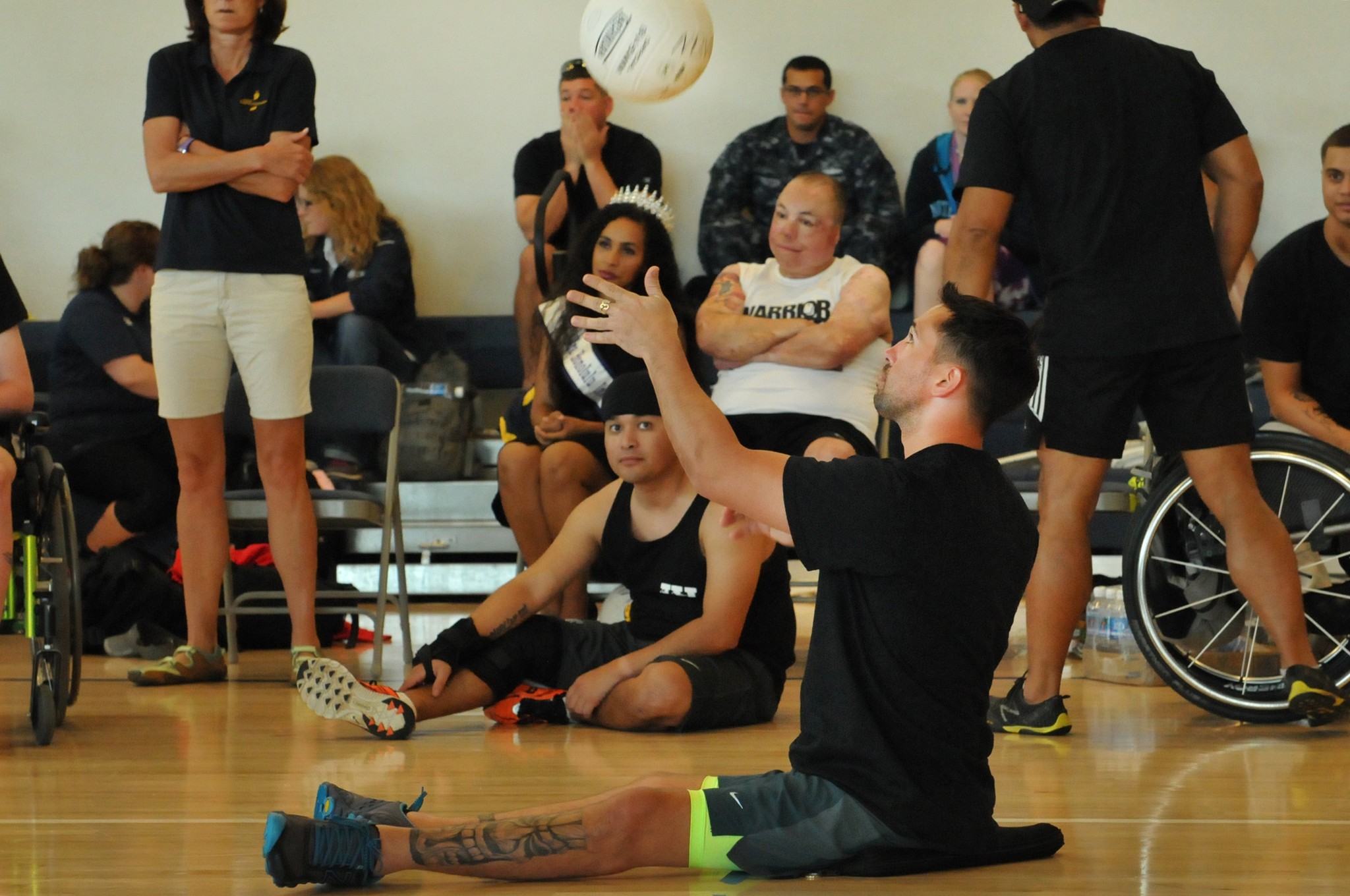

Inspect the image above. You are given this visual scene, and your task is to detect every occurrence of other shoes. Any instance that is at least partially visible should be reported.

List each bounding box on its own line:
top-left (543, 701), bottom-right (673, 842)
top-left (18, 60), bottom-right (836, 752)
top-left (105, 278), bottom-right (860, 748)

top-left (104, 623), bottom-right (173, 659)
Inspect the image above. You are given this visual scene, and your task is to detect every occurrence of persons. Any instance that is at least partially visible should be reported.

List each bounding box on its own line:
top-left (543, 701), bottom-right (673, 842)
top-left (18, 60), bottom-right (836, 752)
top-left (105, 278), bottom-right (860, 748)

top-left (127, 0), bottom-right (322, 686)
top-left (0, 48), bottom-right (1350, 733)
top-left (943, 0), bottom-right (1350, 739)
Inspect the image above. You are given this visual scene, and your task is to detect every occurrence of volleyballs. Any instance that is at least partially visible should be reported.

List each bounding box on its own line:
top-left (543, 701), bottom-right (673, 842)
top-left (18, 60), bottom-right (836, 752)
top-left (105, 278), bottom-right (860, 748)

top-left (576, 0), bottom-right (715, 108)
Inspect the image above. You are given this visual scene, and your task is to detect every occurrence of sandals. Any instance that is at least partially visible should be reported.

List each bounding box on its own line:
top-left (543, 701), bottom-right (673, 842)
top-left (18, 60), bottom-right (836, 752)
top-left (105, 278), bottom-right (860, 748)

top-left (289, 643), bottom-right (323, 689)
top-left (128, 641), bottom-right (229, 686)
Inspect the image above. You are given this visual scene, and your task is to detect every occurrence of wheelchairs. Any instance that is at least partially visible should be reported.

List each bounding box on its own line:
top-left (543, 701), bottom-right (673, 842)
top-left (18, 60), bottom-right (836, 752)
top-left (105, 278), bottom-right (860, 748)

top-left (1122, 430), bottom-right (1350, 722)
top-left (10, 411), bottom-right (81, 748)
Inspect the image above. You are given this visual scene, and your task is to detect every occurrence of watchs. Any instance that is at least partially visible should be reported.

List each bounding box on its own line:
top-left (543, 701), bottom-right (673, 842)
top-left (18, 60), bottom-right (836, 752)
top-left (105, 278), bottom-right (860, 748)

top-left (176, 138), bottom-right (197, 153)
top-left (264, 268), bottom-right (1043, 891)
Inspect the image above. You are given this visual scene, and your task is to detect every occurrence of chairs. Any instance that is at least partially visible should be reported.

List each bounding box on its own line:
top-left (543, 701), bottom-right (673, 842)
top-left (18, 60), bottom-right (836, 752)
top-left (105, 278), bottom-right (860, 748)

top-left (224, 364), bottom-right (414, 681)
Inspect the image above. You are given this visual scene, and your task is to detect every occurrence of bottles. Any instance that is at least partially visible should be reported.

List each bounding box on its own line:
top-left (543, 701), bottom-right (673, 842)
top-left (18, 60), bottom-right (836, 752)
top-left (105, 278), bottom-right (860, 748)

top-left (399, 381), bottom-right (465, 400)
top-left (1085, 586), bottom-right (1145, 678)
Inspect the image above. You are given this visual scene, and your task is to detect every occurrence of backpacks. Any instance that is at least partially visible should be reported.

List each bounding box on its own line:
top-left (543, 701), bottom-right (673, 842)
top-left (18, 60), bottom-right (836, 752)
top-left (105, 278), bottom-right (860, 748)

top-left (379, 344), bottom-right (483, 481)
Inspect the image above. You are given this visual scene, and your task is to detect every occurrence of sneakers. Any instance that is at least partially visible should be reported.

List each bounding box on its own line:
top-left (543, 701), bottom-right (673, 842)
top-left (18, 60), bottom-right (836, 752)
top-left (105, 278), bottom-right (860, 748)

top-left (487, 722), bottom-right (574, 757)
top-left (314, 782), bottom-right (429, 829)
top-left (482, 683), bottom-right (573, 726)
top-left (1282, 660), bottom-right (1350, 729)
top-left (984, 670), bottom-right (1073, 736)
top-left (295, 657), bottom-right (418, 741)
top-left (262, 809), bottom-right (386, 888)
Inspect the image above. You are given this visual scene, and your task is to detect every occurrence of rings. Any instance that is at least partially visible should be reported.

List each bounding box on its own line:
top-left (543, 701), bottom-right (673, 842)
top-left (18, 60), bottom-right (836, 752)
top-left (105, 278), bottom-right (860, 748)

top-left (600, 300), bottom-right (611, 314)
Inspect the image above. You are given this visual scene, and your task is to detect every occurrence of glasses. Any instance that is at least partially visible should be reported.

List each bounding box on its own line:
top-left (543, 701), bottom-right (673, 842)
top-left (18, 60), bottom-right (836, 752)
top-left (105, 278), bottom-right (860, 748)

top-left (560, 58), bottom-right (585, 75)
top-left (784, 85), bottom-right (824, 98)
